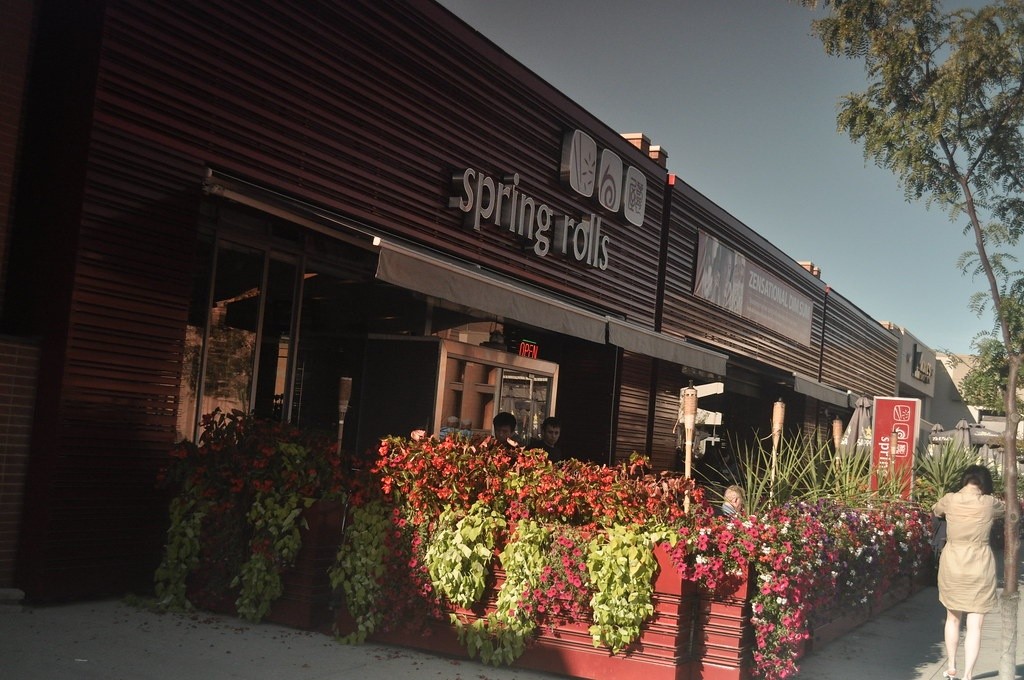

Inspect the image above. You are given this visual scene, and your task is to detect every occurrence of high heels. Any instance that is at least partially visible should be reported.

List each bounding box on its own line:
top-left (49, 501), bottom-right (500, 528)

top-left (943, 668), bottom-right (956, 680)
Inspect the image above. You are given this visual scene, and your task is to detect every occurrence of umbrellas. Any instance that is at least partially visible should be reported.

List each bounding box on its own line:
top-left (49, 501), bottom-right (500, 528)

top-left (925, 417), bottom-right (1020, 480)
top-left (835, 396), bottom-right (872, 461)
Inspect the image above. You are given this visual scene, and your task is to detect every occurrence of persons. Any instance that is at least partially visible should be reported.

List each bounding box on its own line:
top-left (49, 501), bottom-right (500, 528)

top-left (523, 417), bottom-right (561, 464)
top-left (931, 464), bottom-right (1005, 680)
top-left (722, 486), bottom-right (746, 521)
top-left (493, 412), bottom-right (527, 465)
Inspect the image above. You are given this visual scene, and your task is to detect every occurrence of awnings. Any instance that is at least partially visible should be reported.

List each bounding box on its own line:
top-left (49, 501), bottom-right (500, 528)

top-left (687, 339), bottom-right (934, 433)
top-left (211, 170), bottom-right (729, 375)
